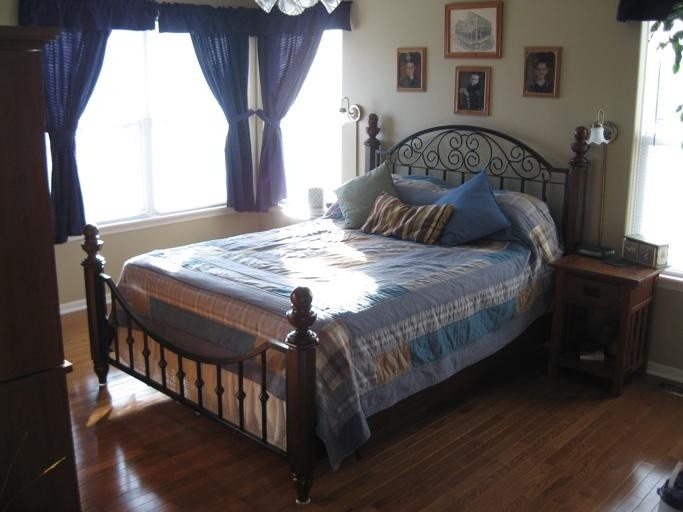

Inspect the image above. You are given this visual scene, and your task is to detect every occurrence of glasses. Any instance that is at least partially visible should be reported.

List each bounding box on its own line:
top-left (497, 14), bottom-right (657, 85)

top-left (575, 109), bottom-right (618, 146)
top-left (339, 97), bottom-right (362, 121)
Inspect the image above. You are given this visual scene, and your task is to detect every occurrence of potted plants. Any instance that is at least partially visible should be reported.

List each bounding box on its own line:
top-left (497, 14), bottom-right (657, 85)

top-left (396, 47), bottom-right (427, 92)
top-left (523, 46), bottom-right (561, 98)
top-left (454, 66), bottom-right (492, 116)
top-left (444, 0), bottom-right (504, 59)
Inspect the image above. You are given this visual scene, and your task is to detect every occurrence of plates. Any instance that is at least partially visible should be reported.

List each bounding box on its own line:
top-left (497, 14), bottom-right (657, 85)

top-left (0, 26), bottom-right (81, 511)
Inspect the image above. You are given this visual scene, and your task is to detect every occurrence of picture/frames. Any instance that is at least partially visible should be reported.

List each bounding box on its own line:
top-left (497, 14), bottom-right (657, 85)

top-left (547, 250), bottom-right (666, 399)
top-left (266, 206), bottom-right (301, 230)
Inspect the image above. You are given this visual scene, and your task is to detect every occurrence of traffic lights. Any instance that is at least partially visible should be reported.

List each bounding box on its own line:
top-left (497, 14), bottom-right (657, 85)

top-left (432, 167), bottom-right (513, 246)
top-left (335, 161), bottom-right (402, 230)
top-left (361, 189), bottom-right (454, 243)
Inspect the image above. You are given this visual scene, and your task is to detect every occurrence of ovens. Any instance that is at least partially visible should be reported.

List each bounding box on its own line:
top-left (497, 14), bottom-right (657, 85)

top-left (80, 112), bottom-right (592, 504)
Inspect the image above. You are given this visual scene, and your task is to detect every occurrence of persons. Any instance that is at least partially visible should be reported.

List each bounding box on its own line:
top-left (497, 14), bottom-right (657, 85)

top-left (460, 74), bottom-right (482, 110)
top-left (399, 57), bottom-right (420, 88)
top-left (528, 58), bottom-right (552, 93)
